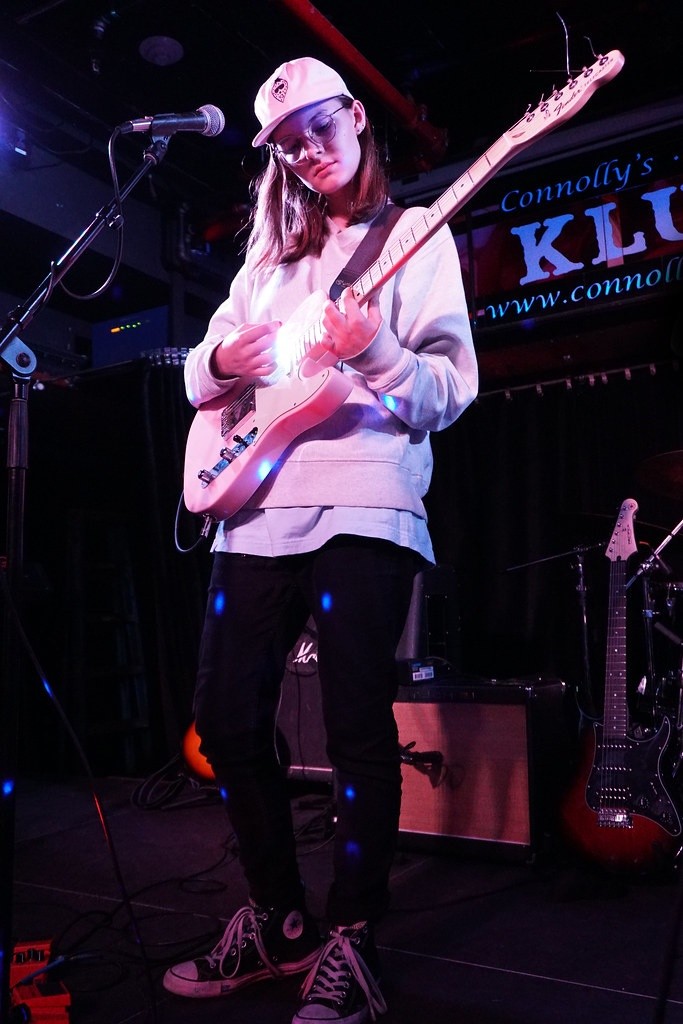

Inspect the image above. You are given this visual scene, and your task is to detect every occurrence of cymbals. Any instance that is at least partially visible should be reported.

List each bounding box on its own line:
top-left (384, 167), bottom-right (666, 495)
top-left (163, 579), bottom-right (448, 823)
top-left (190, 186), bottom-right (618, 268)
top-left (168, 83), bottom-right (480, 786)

top-left (637, 447), bottom-right (683, 505)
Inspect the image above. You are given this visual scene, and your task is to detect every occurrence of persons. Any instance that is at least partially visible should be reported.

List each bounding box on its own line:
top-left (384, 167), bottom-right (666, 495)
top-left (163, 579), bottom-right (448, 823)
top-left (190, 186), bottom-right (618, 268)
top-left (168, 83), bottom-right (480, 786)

top-left (161, 56), bottom-right (478, 1024)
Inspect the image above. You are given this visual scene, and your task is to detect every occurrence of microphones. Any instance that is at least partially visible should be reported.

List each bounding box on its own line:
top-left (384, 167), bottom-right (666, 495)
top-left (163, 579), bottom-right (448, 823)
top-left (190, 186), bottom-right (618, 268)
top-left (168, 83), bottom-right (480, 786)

top-left (654, 552), bottom-right (670, 576)
top-left (401, 750), bottom-right (444, 768)
top-left (117, 105), bottom-right (225, 137)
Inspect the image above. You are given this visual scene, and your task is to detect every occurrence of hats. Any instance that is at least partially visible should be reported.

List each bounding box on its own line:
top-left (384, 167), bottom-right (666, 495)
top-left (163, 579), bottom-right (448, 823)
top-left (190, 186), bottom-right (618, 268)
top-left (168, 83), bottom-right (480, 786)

top-left (251, 57), bottom-right (355, 147)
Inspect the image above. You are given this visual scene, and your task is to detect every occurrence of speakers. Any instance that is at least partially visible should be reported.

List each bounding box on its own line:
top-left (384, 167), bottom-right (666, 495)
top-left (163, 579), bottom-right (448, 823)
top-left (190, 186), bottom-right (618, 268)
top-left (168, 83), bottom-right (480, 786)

top-left (394, 678), bottom-right (581, 852)
top-left (275, 562), bottom-right (461, 779)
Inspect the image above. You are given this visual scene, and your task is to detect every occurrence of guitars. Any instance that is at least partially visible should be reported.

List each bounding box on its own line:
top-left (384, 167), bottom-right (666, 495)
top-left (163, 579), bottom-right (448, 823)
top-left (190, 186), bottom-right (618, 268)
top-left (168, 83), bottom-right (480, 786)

top-left (550, 495), bottom-right (682, 881)
top-left (178, 45), bottom-right (627, 523)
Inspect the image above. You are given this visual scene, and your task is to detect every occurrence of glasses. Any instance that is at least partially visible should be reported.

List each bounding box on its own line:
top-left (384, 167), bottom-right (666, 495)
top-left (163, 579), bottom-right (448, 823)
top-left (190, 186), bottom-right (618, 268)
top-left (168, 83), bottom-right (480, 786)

top-left (277, 106), bottom-right (344, 163)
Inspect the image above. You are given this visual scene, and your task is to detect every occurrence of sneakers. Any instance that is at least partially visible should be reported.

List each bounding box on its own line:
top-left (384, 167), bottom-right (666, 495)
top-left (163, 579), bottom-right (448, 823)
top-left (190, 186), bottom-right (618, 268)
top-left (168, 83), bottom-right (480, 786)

top-left (163, 889), bottom-right (324, 997)
top-left (292, 920), bottom-right (387, 1024)
